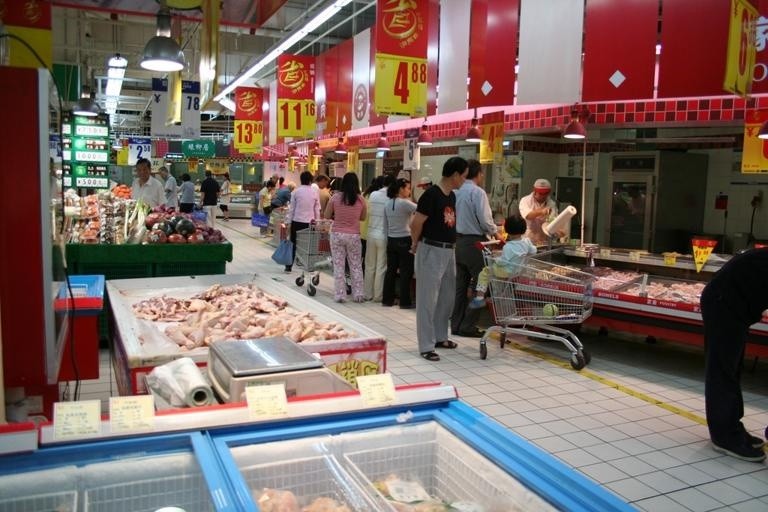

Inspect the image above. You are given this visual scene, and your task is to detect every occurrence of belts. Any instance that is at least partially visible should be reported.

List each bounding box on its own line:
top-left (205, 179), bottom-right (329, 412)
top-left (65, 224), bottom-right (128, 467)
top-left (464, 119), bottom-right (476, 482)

top-left (420, 238), bottom-right (455, 248)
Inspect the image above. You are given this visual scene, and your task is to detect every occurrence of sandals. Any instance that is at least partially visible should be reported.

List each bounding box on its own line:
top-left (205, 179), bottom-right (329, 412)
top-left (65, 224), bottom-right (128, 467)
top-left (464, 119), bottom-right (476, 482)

top-left (420, 350), bottom-right (440, 361)
top-left (435, 339), bottom-right (457, 349)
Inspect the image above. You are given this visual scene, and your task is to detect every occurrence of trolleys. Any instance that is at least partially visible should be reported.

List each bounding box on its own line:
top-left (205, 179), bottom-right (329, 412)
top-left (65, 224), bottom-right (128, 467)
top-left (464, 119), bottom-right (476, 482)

top-left (283, 219), bottom-right (354, 299)
top-left (471, 233), bottom-right (600, 373)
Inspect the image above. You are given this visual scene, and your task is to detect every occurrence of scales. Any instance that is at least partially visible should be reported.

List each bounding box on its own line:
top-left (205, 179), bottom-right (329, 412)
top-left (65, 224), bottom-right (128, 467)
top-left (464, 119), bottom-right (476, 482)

top-left (206, 335), bottom-right (361, 405)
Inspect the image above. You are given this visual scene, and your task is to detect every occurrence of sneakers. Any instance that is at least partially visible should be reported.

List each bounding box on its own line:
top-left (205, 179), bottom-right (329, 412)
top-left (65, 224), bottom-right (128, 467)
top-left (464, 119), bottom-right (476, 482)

top-left (266, 233), bottom-right (274, 237)
top-left (261, 234), bottom-right (266, 238)
top-left (740, 426), bottom-right (764, 448)
top-left (713, 435), bottom-right (766, 461)
top-left (469, 298), bottom-right (486, 308)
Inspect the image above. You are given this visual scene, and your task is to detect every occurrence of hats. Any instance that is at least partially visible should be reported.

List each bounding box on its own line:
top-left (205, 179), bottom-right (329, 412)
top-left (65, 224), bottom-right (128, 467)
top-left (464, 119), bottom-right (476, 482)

top-left (417, 176), bottom-right (431, 187)
top-left (534, 179), bottom-right (552, 194)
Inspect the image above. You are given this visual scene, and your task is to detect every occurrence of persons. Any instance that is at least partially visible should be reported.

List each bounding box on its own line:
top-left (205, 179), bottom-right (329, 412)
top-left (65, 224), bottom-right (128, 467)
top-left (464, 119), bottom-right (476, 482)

top-left (217, 171), bottom-right (232, 221)
top-left (129, 157), bottom-right (167, 210)
top-left (407, 155), bottom-right (471, 361)
top-left (416, 176), bottom-right (434, 190)
top-left (250, 169), bottom-right (424, 310)
top-left (156, 166), bottom-right (178, 213)
top-left (517, 177), bottom-right (567, 248)
top-left (48, 156), bottom-right (59, 201)
top-left (698, 243), bottom-right (767, 463)
top-left (175, 172), bottom-right (196, 213)
top-left (450, 158), bottom-right (507, 339)
top-left (619, 185), bottom-right (646, 222)
top-left (467, 213), bottom-right (537, 310)
top-left (200, 169), bottom-right (221, 229)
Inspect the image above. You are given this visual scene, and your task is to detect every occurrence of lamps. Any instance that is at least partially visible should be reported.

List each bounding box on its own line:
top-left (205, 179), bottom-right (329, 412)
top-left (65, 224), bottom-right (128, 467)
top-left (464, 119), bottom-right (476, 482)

top-left (139, 5), bottom-right (188, 74)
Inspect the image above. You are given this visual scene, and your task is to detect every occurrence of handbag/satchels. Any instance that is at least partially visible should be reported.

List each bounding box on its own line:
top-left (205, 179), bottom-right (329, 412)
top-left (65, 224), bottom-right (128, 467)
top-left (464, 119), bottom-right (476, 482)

top-left (272, 239), bottom-right (293, 265)
top-left (251, 212), bottom-right (269, 227)
top-left (221, 187), bottom-right (233, 195)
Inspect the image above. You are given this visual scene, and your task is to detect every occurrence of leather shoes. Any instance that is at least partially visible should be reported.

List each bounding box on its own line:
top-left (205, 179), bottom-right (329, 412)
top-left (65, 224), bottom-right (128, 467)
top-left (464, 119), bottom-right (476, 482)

top-left (384, 298), bottom-right (398, 306)
top-left (284, 267), bottom-right (292, 272)
top-left (400, 303), bottom-right (415, 308)
top-left (460, 328), bottom-right (485, 337)
